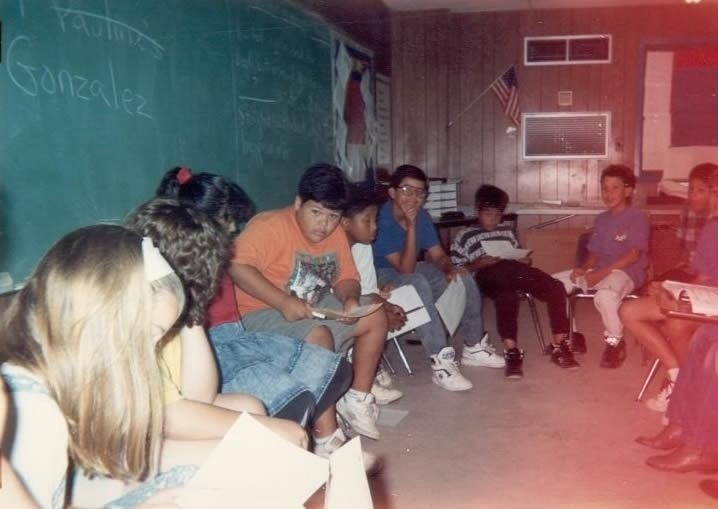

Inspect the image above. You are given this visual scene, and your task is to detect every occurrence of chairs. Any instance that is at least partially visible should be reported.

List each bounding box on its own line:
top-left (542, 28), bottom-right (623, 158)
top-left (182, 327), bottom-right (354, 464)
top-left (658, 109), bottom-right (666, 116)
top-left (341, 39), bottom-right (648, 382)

top-left (568, 232), bottom-right (652, 368)
top-left (446, 229), bottom-right (543, 354)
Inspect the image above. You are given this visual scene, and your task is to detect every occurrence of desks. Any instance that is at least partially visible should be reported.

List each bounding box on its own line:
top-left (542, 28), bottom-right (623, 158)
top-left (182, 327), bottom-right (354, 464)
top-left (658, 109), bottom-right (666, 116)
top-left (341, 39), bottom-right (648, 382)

top-left (428, 212), bottom-right (517, 257)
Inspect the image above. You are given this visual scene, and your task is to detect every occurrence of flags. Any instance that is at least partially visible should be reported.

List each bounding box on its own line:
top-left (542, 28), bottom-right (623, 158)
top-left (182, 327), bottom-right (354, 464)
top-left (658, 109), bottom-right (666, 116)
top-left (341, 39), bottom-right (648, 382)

top-left (333, 40), bottom-right (379, 171)
top-left (490, 65), bottom-right (521, 128)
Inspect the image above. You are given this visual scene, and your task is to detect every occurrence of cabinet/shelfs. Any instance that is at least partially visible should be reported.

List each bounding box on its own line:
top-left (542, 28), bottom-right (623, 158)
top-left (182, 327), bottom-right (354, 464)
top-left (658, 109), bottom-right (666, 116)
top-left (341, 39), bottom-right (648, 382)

top-left (458, 202), bottom-right (686, 230)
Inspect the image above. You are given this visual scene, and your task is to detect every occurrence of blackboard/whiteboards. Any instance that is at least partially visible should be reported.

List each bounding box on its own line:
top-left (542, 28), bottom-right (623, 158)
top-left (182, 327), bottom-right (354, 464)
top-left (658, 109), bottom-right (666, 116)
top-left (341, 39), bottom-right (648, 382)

top-left (1, 0), bottom-right (336, 296)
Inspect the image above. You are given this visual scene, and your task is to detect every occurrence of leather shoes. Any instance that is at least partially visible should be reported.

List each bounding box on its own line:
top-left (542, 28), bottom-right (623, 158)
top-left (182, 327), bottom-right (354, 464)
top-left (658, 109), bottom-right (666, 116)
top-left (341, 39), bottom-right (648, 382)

top-left (646, 447), bottom-right (718, 474)
top-left (635, 424), bottom-right (683, 450)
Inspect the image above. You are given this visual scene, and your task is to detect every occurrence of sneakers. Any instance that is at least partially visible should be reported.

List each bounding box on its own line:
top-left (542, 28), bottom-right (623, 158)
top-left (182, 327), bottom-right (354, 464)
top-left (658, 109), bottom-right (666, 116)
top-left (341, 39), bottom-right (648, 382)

top-left (310, 427), bottom-right (381, 477)
top-left (336, 388), bottom-right (380, 440)
top-left (461, 332), bottom-right (505, 368)
top-left (371, 383), bottom-right (402, 405)
top-left (544, 340), bottom-right (579, 371)
top-left (646, 375), bottom-right (677, 412)
top-left (347, 346), bottom-right (392, 386)
top-left (505, 348), bottom-right (524, 380)
top-left (429, 347), bottom-right (473, 391)
top-left (566, 331), bottom-right (587, 354)
top-left (599, 336), bottom-right (627, 369)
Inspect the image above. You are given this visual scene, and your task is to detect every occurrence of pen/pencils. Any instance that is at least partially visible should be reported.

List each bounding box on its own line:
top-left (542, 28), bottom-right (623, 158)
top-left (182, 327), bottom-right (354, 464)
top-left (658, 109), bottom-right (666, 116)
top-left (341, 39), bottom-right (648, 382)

top-left (312, 312), bottom-right (327, 320)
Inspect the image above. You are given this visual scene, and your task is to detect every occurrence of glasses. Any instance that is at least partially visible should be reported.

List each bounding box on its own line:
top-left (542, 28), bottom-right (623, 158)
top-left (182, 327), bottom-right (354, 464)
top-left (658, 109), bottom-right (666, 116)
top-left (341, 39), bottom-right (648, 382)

top-left (395, 186), bottom-right (425, 200)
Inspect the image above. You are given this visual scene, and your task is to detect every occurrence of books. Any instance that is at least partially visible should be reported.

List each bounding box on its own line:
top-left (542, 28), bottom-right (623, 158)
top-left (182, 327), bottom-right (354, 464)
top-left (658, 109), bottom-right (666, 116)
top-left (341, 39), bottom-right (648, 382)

top-left (661, 278), bottom-right (718, 323)
top-left (174, 410), bottom-right (377, 508)
top-left (302, 303), bottom-right (385, 322)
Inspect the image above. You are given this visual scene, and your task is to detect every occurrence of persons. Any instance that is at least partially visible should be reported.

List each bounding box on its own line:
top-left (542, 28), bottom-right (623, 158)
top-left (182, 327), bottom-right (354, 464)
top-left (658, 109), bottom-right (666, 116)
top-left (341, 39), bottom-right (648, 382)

top-left (449, 184), bottom-right (582, 379)
top-left (617, 169), bottom-right (718, 428)
top-left (229, 163), bottom-right (389, 476)
top-left (119, 198), bottom-right (327, 508)
top-left (370, 164), bottom-right (507, 392)
top-left (667, 163), bottom-right (718, 267)
top-left (698, 479), bottom-right (718, 501)
top-left (0, 371), bottom-right (45, 508)
top-left (338, 184), bottom-right (403, 406)
top-left (0, 224), bottom-right (187, 509)
top-left (634, 323), bottom-right (718, 475)
top-left (155, 167), bottom-right (345, 479)
top-left (545, 164), bottom-right (651, 368)
